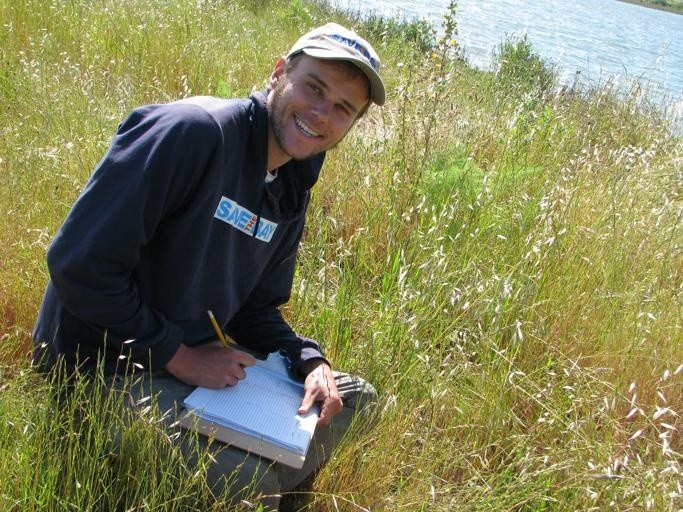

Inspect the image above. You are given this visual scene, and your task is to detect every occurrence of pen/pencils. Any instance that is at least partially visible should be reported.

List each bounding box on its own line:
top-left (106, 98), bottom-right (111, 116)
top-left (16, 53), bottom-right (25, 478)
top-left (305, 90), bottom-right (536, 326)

top-left (207, 310), bottom-right (229, 347)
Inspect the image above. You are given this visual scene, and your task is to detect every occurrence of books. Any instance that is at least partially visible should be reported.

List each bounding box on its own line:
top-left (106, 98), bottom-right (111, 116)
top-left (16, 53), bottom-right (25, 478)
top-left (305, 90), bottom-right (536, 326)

top-left (182, 347), bottom-right (321, 457)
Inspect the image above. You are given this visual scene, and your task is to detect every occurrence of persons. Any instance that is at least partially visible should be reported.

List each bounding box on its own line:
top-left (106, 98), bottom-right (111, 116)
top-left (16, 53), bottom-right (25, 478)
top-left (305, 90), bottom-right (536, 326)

top-left (31, 22), bottom-right (386, 511)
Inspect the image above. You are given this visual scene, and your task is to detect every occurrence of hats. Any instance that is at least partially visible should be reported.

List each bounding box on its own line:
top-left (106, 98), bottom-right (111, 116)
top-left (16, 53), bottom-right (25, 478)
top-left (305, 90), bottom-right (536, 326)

top-left (286, 23), bottom-right (385, 106)
